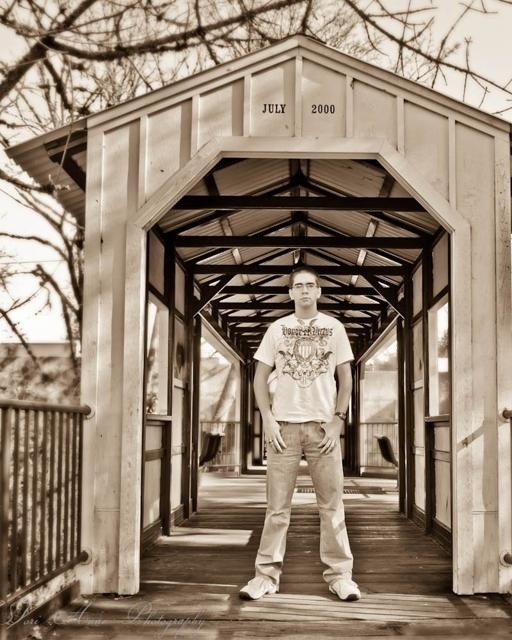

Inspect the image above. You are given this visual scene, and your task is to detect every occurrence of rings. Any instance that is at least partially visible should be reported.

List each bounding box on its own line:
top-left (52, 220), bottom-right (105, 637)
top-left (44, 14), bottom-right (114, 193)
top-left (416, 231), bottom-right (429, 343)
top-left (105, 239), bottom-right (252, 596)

top-left (268, 441), bottom-right (274, 446)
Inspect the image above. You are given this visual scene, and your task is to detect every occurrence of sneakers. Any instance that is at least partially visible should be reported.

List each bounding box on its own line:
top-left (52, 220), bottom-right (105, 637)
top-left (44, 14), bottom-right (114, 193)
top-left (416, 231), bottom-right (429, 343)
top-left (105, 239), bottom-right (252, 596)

top-left (239, 577), bottom-right (276, 600)
top-left (329, 579), bottom-right (361, 602)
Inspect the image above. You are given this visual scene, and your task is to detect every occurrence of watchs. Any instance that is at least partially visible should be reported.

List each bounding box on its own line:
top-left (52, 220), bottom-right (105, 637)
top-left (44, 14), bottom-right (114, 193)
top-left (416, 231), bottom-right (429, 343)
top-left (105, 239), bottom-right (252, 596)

top-left (332, 410), bottom-right (348, 423)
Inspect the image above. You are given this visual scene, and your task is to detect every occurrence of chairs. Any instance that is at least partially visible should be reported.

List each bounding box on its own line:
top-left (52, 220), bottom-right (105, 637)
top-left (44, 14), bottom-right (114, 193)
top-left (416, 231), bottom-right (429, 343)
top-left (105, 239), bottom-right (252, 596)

top-left (375, 434), bottom-right (399, 491)
top-left (201, 431), bottom-right (224, 466)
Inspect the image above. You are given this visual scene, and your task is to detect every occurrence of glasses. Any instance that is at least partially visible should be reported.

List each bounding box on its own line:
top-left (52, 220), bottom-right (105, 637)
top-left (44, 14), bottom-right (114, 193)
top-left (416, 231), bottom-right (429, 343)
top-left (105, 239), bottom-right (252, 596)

top-left (290, 284), bottom-right (319, 292)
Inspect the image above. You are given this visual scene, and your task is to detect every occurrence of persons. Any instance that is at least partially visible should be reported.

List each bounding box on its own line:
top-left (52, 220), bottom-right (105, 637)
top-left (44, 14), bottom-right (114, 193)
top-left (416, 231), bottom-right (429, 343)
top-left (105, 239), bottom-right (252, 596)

top-left (238, 269), bottom-right (361, 602)
